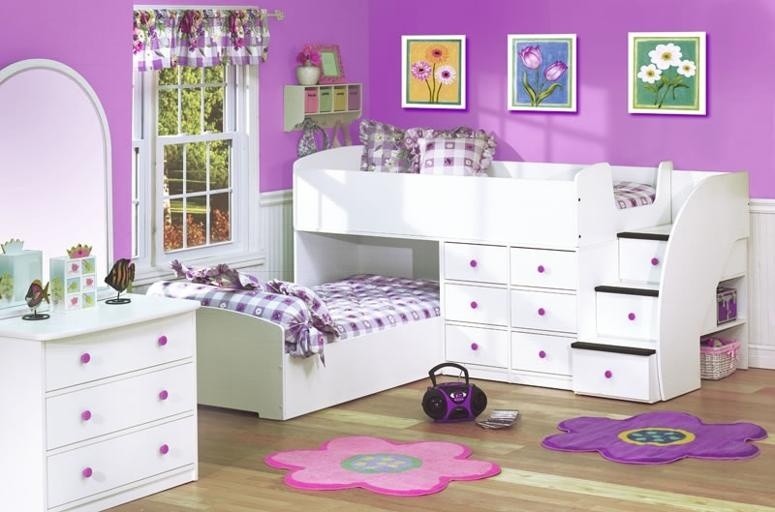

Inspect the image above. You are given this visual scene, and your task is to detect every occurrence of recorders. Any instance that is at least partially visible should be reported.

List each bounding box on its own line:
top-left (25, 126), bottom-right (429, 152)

top-left (422, 363), bottom-right (487, 423)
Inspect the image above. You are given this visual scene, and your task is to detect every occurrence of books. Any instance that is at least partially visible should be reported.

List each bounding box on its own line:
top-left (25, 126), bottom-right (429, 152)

top-left (476, 409), bottom-right (521, 430)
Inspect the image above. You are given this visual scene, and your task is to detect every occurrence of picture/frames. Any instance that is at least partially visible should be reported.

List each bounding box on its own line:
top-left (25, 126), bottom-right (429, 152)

top-left (400, 35), bottom-right (467, 111)
top-left (508, 34), bottom-right (577, 112)
top-left (627, 32), bottom-right (707, 114)
top-left (316, 45), bottom-right (347, 84)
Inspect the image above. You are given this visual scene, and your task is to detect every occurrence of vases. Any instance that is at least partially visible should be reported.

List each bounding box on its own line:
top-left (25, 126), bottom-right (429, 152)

top-left (296, 66), bottom-right (320, 84)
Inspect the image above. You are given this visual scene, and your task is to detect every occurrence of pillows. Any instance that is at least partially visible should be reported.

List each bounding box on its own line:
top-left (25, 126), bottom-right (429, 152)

top-left (170, 259), bottom-right (341, 366)
top-left (360, 120), bottom-right (495, 177)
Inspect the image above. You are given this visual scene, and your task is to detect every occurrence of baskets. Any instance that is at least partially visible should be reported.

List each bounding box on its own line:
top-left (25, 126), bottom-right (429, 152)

top-left (700, 339), bottom-right (740, 379)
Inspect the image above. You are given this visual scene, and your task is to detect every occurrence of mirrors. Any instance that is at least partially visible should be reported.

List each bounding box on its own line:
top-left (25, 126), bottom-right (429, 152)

top-left (0, 58), bottom-right (115, 320)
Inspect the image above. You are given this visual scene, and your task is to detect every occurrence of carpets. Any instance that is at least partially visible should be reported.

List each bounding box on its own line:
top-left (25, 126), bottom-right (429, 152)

top-left (263, 436), bottom-right (501, 497)
top-left (541, 412), bottom-right (767, 465)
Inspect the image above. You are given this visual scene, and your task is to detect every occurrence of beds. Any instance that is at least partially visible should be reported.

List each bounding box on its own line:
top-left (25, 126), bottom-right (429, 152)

top-left (146, 273), bottom-right (440, 421)
top-left (292, 145), bottom-right (751, 402)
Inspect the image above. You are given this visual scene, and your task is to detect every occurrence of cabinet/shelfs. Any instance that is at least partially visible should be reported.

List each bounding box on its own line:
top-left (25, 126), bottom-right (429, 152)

top-left (439, 241), bottom-right (575, 392)
top-left (0, 293), bottom-right (201, 512)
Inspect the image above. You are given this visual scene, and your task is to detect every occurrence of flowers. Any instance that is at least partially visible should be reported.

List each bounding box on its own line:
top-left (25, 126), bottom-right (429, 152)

top-left (295, 45), bottom-right (320, 66)
